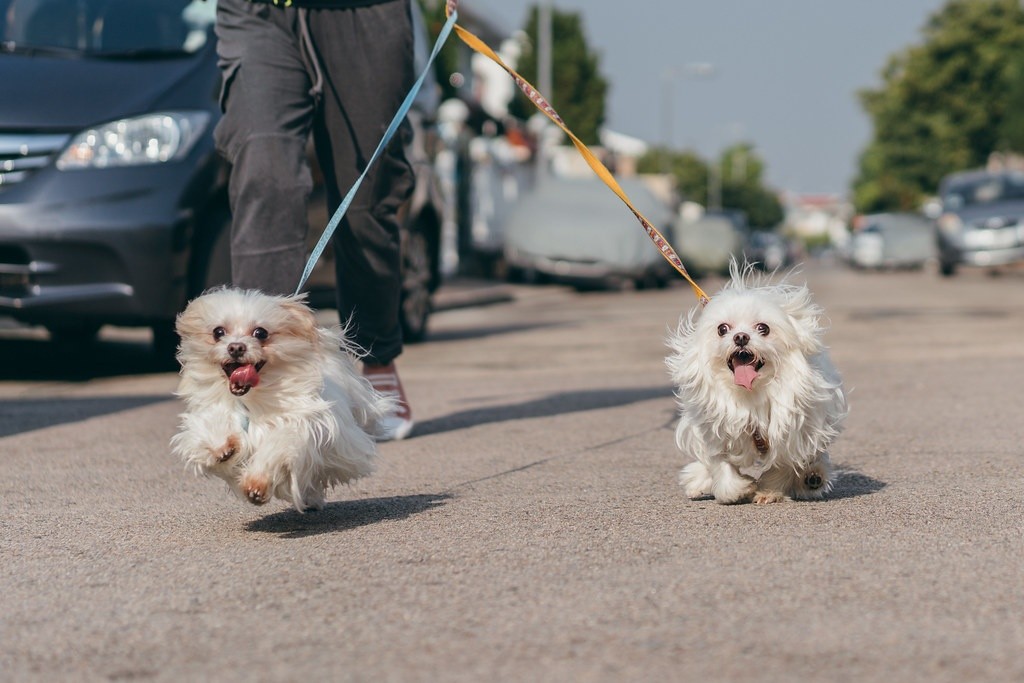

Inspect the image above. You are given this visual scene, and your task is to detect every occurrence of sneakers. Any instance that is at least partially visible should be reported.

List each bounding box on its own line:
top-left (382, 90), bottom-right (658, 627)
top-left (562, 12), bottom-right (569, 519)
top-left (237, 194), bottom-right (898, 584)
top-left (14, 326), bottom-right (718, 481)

top-left (362, 362), bottom-right (414, 441)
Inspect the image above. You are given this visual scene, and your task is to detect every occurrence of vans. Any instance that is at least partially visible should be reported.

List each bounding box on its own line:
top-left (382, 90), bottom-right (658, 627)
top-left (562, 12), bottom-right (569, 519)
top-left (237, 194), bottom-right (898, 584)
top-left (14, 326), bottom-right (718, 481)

top-left (1, 1), bottom-right (444, 356)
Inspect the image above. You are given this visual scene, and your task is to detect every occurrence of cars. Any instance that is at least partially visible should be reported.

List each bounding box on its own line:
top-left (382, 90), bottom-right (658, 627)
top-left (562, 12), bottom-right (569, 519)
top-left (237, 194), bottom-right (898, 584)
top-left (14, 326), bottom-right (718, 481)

top-left (675, 208), bottom-right (751, 278)
top-left (921, 170), bottom-right (1023, 276)
top-left (834, 211), bottom-right (934, 272)
top-left (499, 176), bottom-right (680, 293)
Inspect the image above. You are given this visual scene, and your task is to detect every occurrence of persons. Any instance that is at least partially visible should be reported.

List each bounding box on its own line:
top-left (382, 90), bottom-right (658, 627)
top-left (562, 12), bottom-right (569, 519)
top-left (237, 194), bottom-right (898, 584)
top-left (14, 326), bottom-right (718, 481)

top-left (208, 0), bottom-right (419, 444)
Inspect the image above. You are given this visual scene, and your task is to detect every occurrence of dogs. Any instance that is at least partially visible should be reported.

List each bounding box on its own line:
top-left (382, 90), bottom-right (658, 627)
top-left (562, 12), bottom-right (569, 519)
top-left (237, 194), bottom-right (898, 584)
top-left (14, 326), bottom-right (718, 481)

top-left (661, 251), bottom-right (852, 505)
top-left (166, 285), bottom-right (404, 515)
